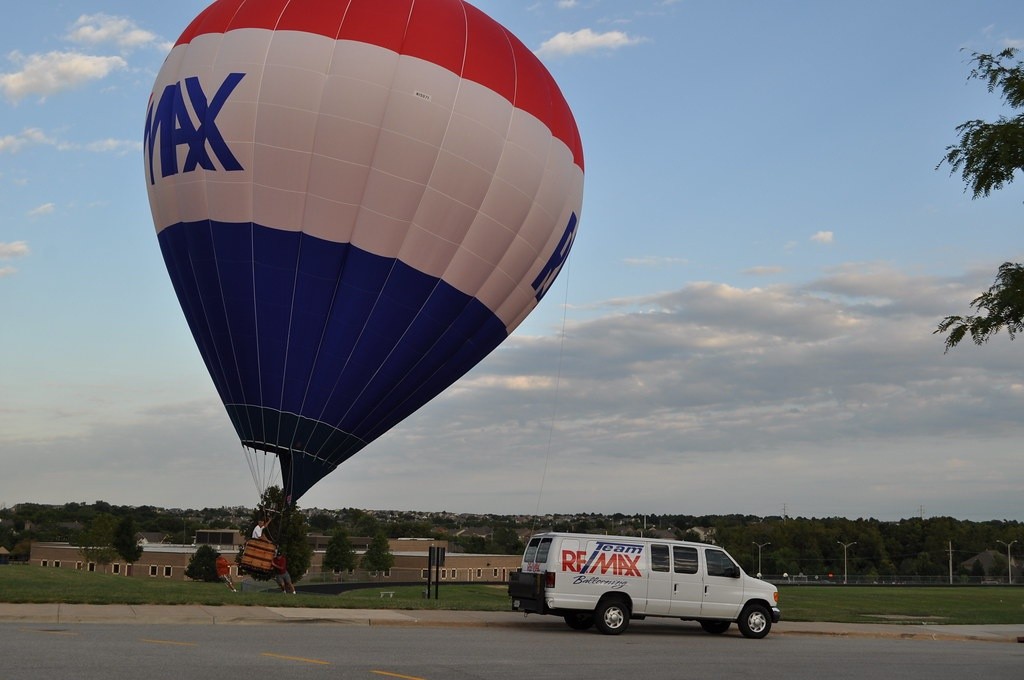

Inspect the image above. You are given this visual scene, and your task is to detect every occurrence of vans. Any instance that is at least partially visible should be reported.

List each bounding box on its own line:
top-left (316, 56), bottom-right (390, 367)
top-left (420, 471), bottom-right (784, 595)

top-left (510, 530), bottom-right (783, 641)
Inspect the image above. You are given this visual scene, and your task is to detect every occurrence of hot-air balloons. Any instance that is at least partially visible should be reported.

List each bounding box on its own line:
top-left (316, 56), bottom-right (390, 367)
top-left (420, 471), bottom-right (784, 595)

top-left (141, 1), bottom-right (586, 582)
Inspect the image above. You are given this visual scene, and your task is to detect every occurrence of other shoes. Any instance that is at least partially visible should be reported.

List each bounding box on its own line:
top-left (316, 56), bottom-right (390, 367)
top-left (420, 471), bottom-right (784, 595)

top-left (283, 591), bottom-right (286, 594)
top-left (230, 589), bottom-right (237, 593)
top-left (293, 591), bottom-right (296, 595)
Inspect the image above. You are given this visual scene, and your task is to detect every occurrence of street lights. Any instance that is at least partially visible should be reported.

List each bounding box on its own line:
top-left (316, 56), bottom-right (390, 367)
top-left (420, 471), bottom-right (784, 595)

top-left (996, 539), bottom-right (1018, 585)
top-left (837, 540), bottom-right (857, 584)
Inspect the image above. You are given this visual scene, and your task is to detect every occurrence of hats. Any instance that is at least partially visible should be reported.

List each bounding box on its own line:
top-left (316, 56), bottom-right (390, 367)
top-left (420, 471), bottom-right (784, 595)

top-left (276, 552), bottom-right (282, 556)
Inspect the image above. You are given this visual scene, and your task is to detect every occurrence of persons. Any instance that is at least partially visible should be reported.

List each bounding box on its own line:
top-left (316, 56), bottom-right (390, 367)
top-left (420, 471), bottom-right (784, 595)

top-left (272, 551), bottom-right (297, 595)
top-left (251, 518), bottom-right (274, 543)
top-left (216, 553), bottom-right (239, 592)
